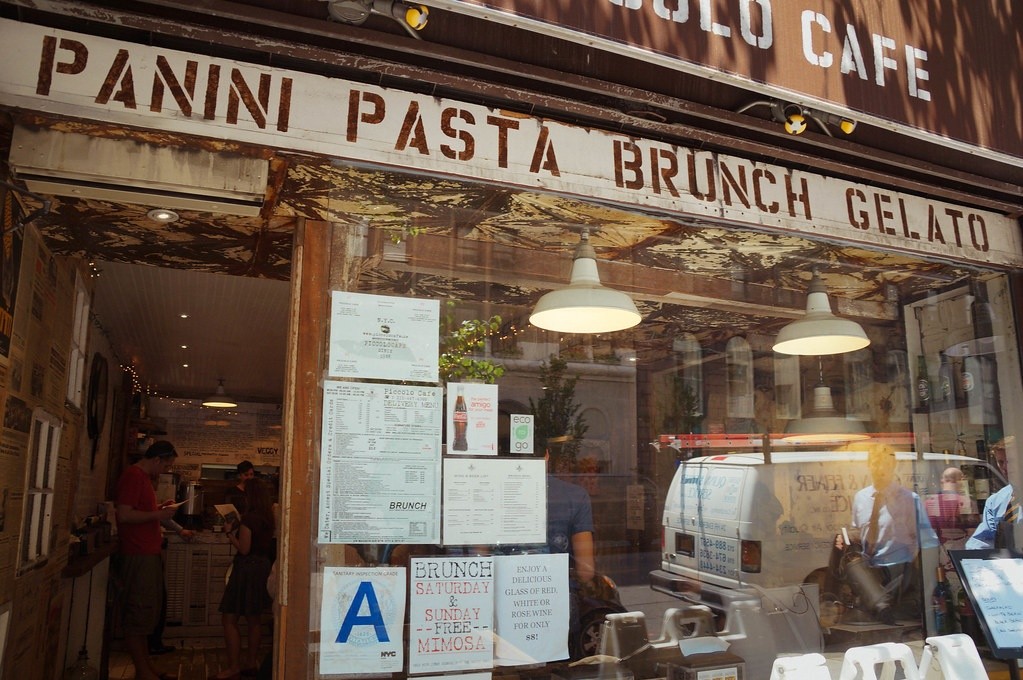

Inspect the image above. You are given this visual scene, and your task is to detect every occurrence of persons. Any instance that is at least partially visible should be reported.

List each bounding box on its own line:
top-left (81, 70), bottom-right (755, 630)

top-left (222, 461), bottom-right (256, 514)
top-left (835, 449), bottom-right (941, 621)
top-left (266, 398), bottom-right (595, 665)
top-left (990, 434), bottom-right (1023, 559)
top-left (923, 467), bottom-right (983, 646)
top-left (114, 438), bottom-right (198, 680)
top-left (210, 477), bottom-right (277, 680)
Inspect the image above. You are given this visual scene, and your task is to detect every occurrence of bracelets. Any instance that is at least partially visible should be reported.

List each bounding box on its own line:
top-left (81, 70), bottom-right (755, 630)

top-left (226, 531), bottom-right (231, 538)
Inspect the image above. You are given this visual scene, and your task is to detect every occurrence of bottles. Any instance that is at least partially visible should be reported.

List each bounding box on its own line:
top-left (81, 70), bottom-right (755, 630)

top-left (939, 350), bottom-right (955, 410)
top-left (83, 513), bottom-right (111, 553)
top-left (928, 452), bottom-right (939, 494)
top-left (959, 449), bottom-right (980, 527)
top-left (933, 567), bottom-right (954, 636)
top-left (958, 590), bottom-right (984, 646)
top-left (453, 387), bottom-right (468, 451)
top-left (917, 355), bottom-right (931, 413)
top-left (975, 440), bottom-right (992, 514)
top-left (971, 285), bottom-right (994, 356)
top-left (943, 450), bottom-right (954, 469)
top-left (64, 650), bottom-right (98, 680)
top-left (960, 346), bottom-right (981, 408)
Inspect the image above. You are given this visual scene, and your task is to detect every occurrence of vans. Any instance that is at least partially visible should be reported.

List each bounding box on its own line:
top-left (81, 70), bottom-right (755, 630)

top-left (649, 432), bottom-right (1009, 612)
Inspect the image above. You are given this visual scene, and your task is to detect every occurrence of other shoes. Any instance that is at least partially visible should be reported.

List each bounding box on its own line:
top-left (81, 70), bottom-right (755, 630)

top-left (242, 665), bottom-right (258, 676)
top-left (159, 673), bottom-right (178, 680)
top-left (208, 668), bottom-right (241, 680)
top-left (149, 644), bottom-right (175, 655)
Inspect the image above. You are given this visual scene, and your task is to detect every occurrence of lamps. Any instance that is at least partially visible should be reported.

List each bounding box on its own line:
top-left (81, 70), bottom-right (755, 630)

top-left (328, 0), bottom-right (430, 42)
top-left (772, 266), bottom-right (871, 355)
top-left (202, 379), bottom-right (238, 407)
top-left (944, 272), bottom-right (1012, 354)
top-left (784, 354), bottom-right (869, 444)
top-left (528, 225), bottom-right (642, 334)
top-left (738, 98), bottom-right (858, 137)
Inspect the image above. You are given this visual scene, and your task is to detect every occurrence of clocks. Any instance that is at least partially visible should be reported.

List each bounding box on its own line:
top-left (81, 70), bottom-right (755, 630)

top-left (89, 353), bottom-right (109, 437)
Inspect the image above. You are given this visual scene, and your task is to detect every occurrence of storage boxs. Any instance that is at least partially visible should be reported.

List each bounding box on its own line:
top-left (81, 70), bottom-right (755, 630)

top-left (68, 521), bottom-right (113, 558)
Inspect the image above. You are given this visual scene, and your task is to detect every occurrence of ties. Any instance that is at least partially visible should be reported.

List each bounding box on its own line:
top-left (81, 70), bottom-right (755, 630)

top-left (1004, 491), bottom-right (1020, 526)
top-left (867, 491), bottom-right (881, 559)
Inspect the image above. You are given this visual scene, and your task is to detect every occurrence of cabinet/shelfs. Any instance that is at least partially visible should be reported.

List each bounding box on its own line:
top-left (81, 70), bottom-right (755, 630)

top-left (899, 276), bottom-right (1013, 664)
